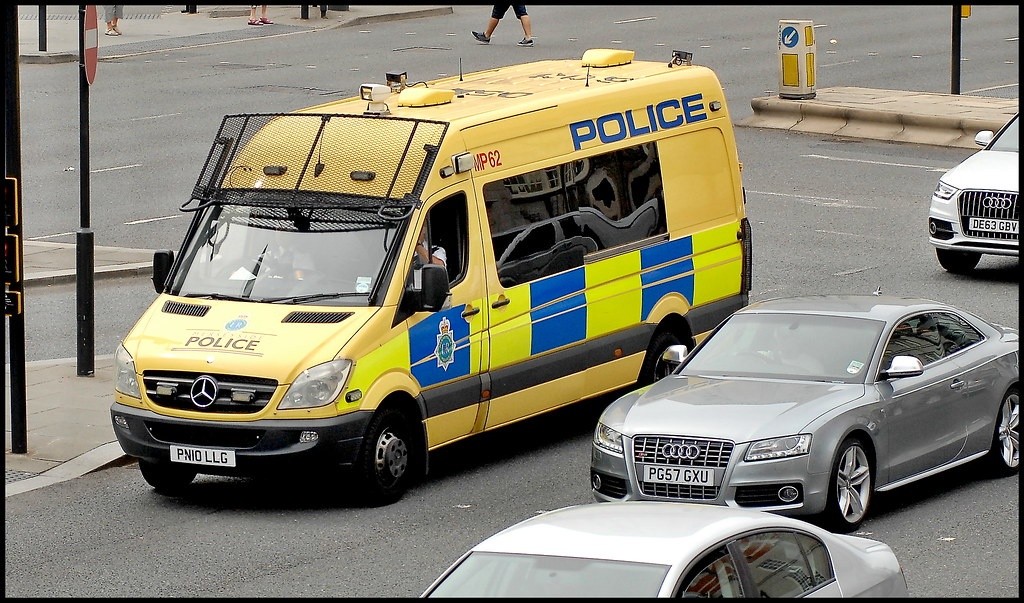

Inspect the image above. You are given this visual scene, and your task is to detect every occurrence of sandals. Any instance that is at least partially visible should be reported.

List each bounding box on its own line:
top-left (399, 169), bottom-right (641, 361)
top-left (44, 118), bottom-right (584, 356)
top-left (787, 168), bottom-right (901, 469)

top-left (248, 18), bottom-right (274, 26)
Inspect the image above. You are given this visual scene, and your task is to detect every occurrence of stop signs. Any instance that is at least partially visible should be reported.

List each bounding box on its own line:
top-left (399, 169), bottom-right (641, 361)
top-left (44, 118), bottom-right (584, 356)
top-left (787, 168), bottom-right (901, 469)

top-left (82, 6), bottom-right (99, 83)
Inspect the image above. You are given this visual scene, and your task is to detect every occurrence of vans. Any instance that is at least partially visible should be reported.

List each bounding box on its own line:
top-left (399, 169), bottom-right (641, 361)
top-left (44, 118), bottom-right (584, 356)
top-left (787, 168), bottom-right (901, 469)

top-left (109, 47), bottom-right (754, 506)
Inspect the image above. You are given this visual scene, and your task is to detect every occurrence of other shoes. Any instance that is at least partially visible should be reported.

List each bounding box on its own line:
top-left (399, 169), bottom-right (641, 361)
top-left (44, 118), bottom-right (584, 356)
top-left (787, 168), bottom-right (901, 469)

top-left (180, 10), bottom-right (198, 16)
top-left (104, 25), bottom-right (122, 36)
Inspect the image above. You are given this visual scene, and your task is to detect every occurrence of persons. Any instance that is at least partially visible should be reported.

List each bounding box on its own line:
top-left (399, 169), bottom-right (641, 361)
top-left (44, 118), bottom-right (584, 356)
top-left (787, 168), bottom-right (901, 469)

top-left (248, 5), bottom-right (274, 25)
top-left (103, 6), bottom-right (123, 35)
top-left (471, 5), bottom-right (535, 46)
top-left (245, 230), bottom-right (315, 281)
top-left (378, 220), bottom-right (447, 289)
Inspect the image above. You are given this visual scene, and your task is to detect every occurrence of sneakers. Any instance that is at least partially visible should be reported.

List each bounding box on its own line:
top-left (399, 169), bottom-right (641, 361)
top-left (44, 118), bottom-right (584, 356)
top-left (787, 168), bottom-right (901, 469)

top-left (471, 31), bottom-right (491, 44)
top-left (517, 37), bottom-right (534, 47)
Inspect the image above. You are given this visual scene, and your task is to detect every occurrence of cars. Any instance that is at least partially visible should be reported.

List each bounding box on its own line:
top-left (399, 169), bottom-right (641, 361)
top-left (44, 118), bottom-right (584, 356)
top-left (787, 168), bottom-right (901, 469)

top-left (590, 296), bottom-right (1018, 531)
top-left (926, 112), bottom-right (1019, 276)
top-left (418, 501), bottom-right (909, 601)
